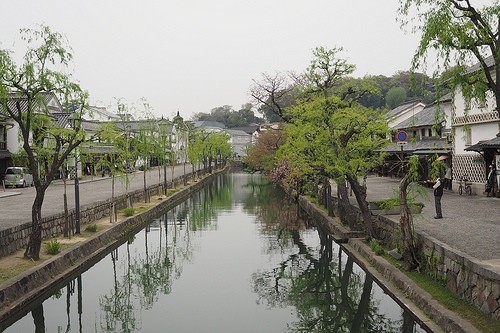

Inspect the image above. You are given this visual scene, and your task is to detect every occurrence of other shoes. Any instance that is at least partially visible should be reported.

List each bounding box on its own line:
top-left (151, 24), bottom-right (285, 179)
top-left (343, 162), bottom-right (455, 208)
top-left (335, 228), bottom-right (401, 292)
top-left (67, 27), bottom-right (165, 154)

top-left (434, 215), bottom-right (442, 219)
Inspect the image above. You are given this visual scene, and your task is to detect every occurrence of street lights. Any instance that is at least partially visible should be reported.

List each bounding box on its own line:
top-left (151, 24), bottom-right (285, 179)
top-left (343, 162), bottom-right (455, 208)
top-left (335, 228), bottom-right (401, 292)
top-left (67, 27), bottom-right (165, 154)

top-left (67, 110), bottom-right (83, 234)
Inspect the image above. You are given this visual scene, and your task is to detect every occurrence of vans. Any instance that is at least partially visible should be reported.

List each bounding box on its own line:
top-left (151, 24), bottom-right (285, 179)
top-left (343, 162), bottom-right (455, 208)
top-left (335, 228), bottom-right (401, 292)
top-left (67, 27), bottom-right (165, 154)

top-left (2, 166), bottom-right (35, 187)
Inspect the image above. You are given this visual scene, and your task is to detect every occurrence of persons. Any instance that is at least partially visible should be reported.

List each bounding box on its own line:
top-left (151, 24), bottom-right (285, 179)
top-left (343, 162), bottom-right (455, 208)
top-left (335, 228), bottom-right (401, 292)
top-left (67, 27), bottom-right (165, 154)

top-left (442, 164), bottom-right (452, 192)
top-left (432, 175), bottom-right (445, 219)
top-left (484, 164), bottom-right (496, 198)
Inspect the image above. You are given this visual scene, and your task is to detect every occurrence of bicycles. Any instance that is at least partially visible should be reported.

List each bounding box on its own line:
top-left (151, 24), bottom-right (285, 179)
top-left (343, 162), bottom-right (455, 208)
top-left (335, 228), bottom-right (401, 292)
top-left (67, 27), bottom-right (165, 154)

top-left (458, 178), bottom-right (473, 196)
